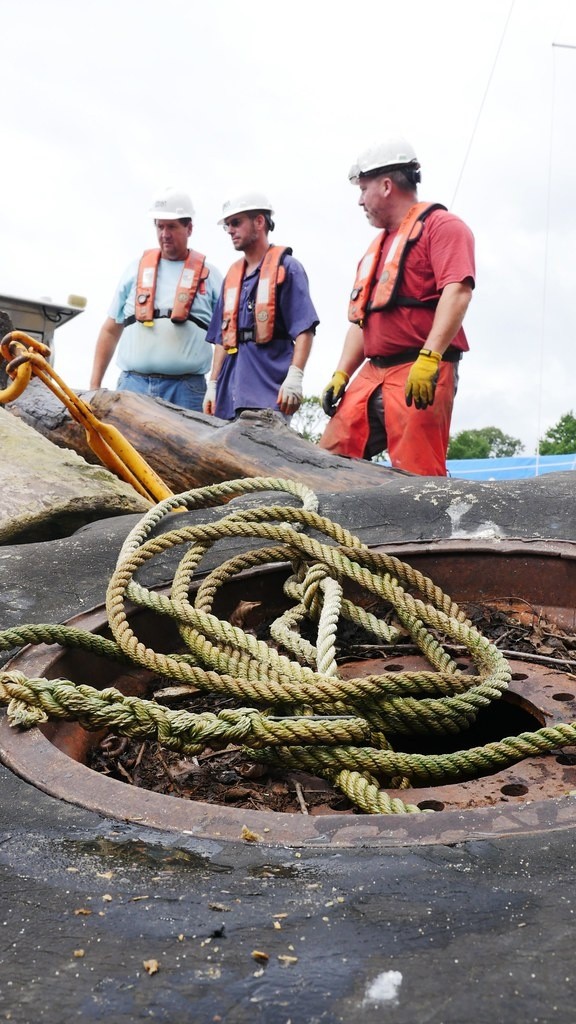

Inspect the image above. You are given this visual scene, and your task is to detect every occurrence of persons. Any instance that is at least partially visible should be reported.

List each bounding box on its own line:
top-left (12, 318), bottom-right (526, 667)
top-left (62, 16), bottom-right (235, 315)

top-left (318, 138), bottom-right (475, 476)
top-left (90, 191), bottom-right (224, 413)
top-left (202, 193), bottom-right (320, 425)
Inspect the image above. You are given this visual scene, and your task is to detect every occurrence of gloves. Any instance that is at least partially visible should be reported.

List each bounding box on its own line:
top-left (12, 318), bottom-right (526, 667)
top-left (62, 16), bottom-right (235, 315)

top-left (321, 370), bottom-right (349, 417)
top-left (404, 348), bottom-right (443, 410)
top-left (276, 365), bottom-right (304, 416)
top-left (203, 381), bottom-right (218, 416)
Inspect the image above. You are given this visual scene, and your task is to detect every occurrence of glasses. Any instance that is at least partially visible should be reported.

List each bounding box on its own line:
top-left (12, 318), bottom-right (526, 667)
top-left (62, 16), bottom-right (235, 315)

top-left (222, 217), bottom-right (259, 231)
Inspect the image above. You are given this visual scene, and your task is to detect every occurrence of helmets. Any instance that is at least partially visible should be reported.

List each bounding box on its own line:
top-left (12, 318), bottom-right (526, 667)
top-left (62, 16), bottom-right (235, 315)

top-left (148, 188), bottom-right (195, 220)
top-left (347, 135), bottom-right (421, 185)
top-left (216, 192), bottom-right (276, 226)
